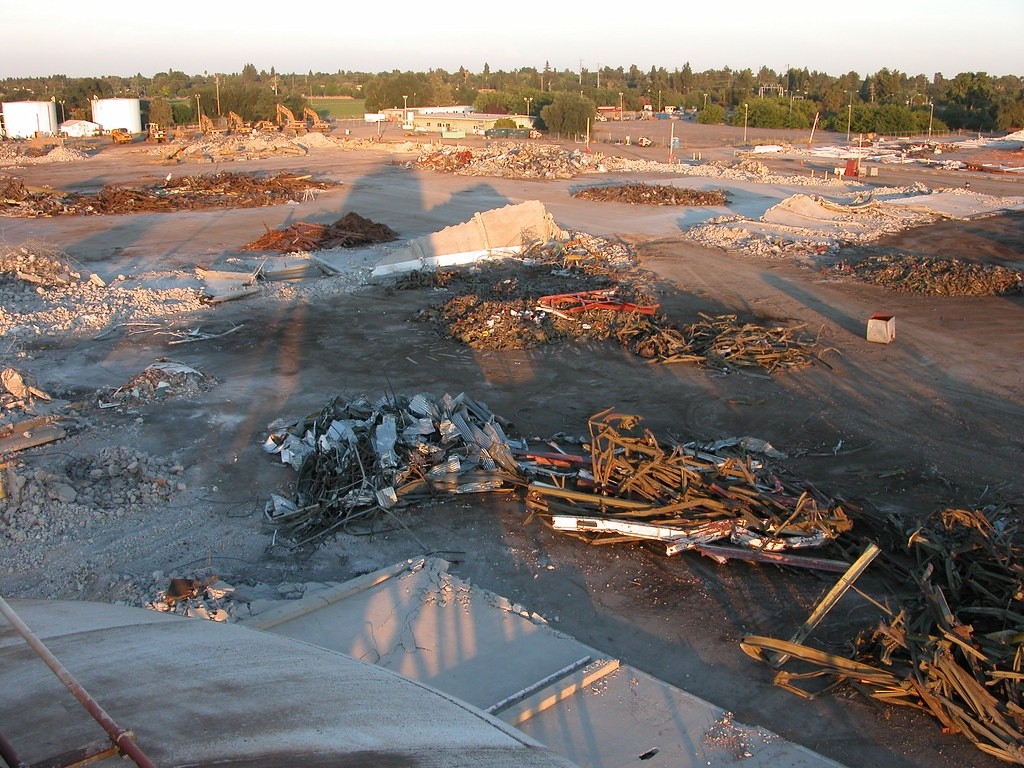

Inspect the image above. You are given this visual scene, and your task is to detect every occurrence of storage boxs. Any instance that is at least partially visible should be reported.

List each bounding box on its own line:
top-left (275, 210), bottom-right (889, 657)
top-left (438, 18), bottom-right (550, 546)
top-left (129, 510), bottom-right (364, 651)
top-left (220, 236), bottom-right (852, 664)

top-left (834, 160), bottom-right (878, 176)
top-left (866, 311), bottom-right (896, 343)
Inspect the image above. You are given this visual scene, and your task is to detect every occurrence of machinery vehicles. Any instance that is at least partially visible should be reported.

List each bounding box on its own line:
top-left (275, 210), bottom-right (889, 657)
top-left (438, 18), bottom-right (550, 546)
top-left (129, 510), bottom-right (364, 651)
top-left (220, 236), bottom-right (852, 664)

top-left (303, 108), bottom-right (332, 134)
top-left (200, 113), bottom-right (224, 142)
top-left (276, 103), bottom-right (307, 138)
top-left (145, 122), bottom-right (168, 144)
top-left (226, 110), bottom-right (276, 135)
top-left (174, 125), bottom-right (191, 142)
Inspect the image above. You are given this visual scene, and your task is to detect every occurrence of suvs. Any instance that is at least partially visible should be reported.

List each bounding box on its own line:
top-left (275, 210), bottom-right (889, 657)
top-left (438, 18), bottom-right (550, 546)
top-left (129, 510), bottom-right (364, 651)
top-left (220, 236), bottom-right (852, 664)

top-left (111, 128), bottom-right (133, 144)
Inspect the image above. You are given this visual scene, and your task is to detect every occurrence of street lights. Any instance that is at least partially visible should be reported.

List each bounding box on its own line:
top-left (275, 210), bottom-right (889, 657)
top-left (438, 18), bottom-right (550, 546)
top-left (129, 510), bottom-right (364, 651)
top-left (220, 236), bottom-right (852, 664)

top-left (928, 104), bottom-right (934, 140)
top-left (659, 90), bottom-right (662, 110)
top-left (703, 93), bottom-right (708, 110)
top-left (524, 97), bottom-right (533, 116)
top-left (195, 94), bottom-right (202, 131)
top-left (844, 89), bottom-right (860, 106)
top-left (884, 94), bottom-right (895, 101)
top-left (846, 105), bottom-right (851, 142)
top-left (910, 94), bottom-right (921, 111)
top-left (619, 93), bottom-right (624, 122)
top-left (743, 103), bottom-right (748, 141)
top-left (59, 100), bottom-right (66, 122)
top-left (403, 95), bottom-right (408, 125)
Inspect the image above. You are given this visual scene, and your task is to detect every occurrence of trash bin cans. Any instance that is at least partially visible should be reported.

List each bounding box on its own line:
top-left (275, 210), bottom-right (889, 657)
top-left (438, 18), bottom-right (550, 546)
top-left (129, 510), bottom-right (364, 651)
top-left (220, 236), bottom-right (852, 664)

top-left (673, 137), bottom-right (679, 148)
